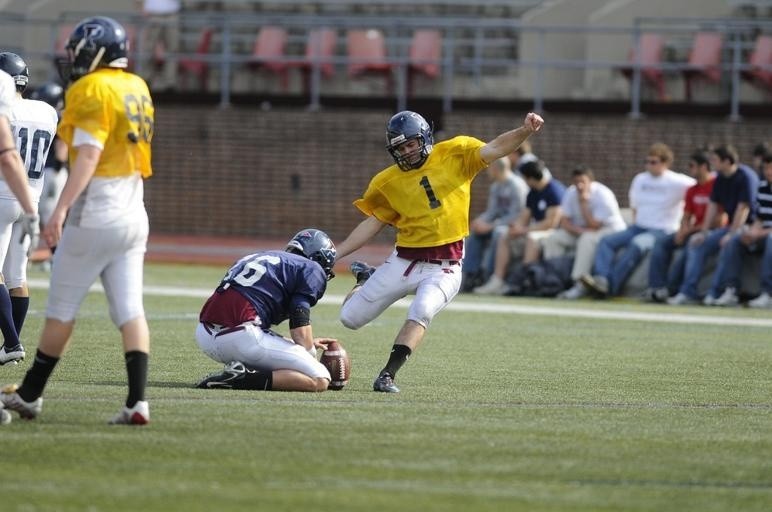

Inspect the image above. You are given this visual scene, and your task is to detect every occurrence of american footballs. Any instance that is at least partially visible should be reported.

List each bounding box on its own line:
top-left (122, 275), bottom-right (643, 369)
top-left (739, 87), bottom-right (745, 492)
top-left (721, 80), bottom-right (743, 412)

top-left (320, 342), bottom-right (350, 391)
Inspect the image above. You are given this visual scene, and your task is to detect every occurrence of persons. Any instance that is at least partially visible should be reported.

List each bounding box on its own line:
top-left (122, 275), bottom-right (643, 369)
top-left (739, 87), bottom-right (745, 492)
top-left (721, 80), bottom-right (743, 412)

top-left (193, 224), bottom-right (338, 395)
top-left (455, 141), bottom-right (628, 300)
top-left (1, 13), bottom-right (156, 427)
top-left (581, 142), bottom-right (772, 307)
top-left (1, 53), bottom-right (67, 362)
top-left (332, 110), bottom-right (546, 395)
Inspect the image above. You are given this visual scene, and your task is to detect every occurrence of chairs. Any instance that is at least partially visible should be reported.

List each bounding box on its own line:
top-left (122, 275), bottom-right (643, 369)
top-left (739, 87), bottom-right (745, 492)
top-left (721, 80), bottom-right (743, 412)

top-left (159, 28), bottom-right (217, 92)
top-left (678, 28), bottom-right (726, 92)
top-left (733, 28), bottom-right (772, 93)
top-left (405, 26), bottom-right (444, 91)
top-left (294, 26), bottom-right (339, 93)
top-left (611, 26), bottom-right (669, 93)
top-left (345, 28), bottom-right (400, 93)
top-left (250, 28), bottom-right (291, 93)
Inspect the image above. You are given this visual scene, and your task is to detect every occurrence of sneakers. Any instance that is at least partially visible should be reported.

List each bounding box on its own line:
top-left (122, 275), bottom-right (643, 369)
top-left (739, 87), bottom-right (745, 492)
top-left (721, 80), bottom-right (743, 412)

top-left (0, 345), bottom-right (25, 363)
top-left (351, 261), bottom-right (377, 283)
top-left (195, 360), bottom-right (256, 387)
top-left (473, 274), bottom-right (772, 308)
top-left (374, 371), bottom-right (399, 392)
top-left (0, 386), bottom-right (42, 425)
top-left (107, 401), bottom-right (150, 424)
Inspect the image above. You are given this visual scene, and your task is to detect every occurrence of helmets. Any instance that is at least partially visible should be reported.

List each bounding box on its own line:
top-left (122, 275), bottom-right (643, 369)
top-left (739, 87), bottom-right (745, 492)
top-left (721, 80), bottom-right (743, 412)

top-left (384, 111), bottom-right (432, 171)
top-left (287, 228), bottom-right (336, 281)
top-left (0, 15), bottom-right (130, 92)
